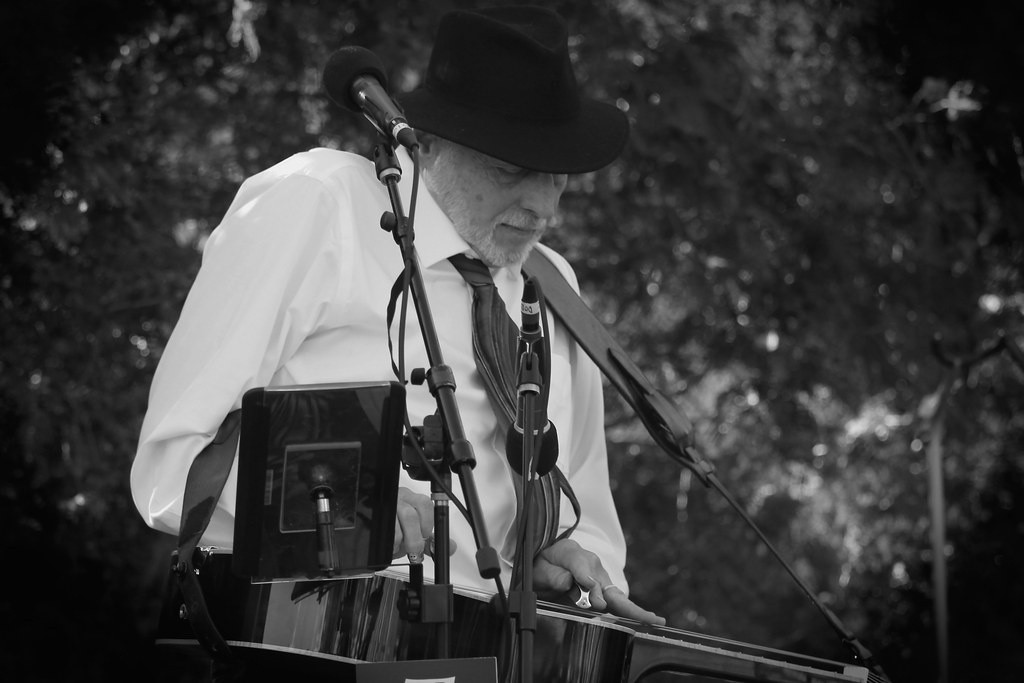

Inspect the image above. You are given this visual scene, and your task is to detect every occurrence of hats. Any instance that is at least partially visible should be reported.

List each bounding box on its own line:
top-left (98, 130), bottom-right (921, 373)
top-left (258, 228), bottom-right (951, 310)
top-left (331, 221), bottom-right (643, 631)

top-left (394, 7), bottom-right (629, 174)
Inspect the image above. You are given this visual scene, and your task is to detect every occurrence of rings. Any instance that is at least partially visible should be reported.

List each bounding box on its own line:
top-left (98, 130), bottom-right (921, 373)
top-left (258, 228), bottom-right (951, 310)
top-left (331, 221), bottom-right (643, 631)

top-left (601, 584), bottom-right (618, 593)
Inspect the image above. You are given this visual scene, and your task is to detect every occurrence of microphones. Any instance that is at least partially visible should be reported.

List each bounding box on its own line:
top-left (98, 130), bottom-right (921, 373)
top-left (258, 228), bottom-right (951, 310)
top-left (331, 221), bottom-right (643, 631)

top-left (504, 280), bottom-right (559, 477)
top-left (326, 44), bottom-right (420, 152)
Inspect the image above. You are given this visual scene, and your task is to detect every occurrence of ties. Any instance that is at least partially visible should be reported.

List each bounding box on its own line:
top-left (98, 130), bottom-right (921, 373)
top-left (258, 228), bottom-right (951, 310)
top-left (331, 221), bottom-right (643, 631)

top-left (448, 253), bottom-right (581, 585)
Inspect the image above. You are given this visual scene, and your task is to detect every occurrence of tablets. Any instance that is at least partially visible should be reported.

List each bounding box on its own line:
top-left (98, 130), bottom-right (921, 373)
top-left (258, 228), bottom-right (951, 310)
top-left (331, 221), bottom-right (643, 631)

top-left (231, 380), bottom-right (406, 580)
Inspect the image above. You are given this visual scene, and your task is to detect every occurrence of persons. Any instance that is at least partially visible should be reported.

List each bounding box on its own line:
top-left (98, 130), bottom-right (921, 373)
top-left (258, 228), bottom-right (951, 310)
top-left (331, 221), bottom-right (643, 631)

top-left (128, 0), bottom-right (666, 683)
